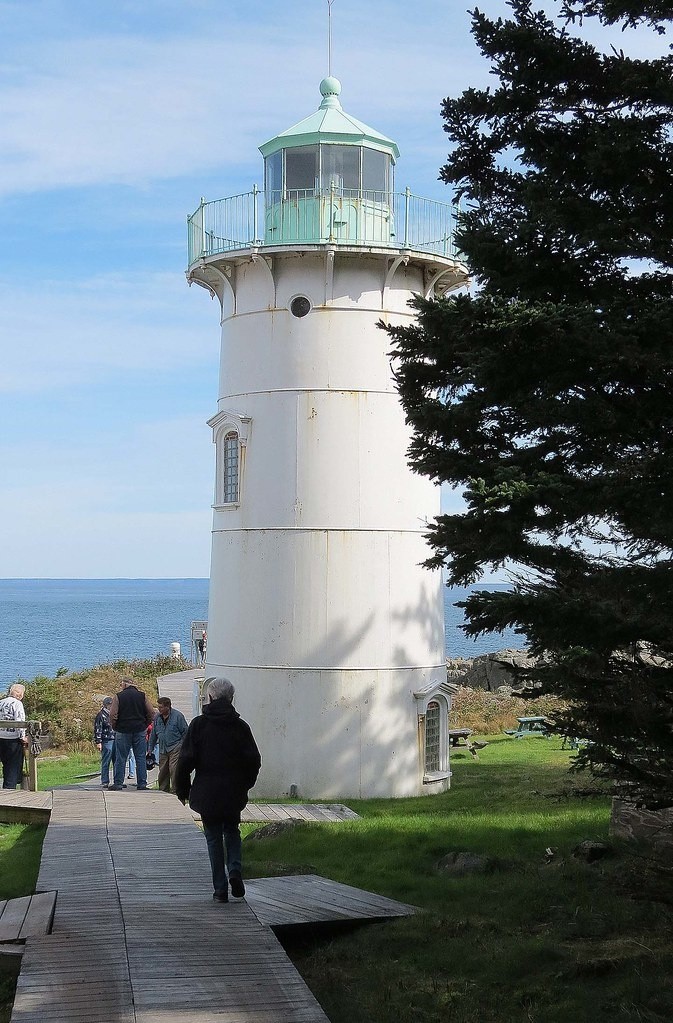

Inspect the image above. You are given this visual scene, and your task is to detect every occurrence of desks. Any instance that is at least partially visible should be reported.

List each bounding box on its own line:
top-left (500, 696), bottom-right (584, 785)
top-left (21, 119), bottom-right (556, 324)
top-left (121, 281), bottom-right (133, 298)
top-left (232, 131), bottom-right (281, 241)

top-left (516, 716), bottom-right (548, 739)
top-left (448, 728), bottom-right (479, 761)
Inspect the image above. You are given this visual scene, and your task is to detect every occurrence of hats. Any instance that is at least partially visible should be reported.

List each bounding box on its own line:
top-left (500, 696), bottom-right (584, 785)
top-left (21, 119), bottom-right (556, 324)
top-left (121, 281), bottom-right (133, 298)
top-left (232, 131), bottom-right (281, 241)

top-left (146, 753), bottom-right (156, 770)
top-left (122, 675), bottom-right (137, 687)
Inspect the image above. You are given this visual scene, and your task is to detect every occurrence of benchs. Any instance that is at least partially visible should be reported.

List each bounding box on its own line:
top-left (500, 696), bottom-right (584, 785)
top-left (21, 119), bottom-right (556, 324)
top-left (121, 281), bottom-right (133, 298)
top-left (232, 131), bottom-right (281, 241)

top-left (505, 729), bottom-right (546, 736)
top-left (474, 739), bottom-right (490, 747)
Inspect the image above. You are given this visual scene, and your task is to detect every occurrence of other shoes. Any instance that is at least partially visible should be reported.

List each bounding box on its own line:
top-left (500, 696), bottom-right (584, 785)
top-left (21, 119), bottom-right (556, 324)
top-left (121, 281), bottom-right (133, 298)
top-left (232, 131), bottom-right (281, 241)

top-left (229, 869), bottom-right (246, 898)
top-left (128, 776), bottom-right (135, 779)
top-left (137, 785), bottom-right (146, 790)
top-left (122, 784), bottom-right (127, 788)
top-left (102, 782), bottom-right (108, 788)
top-left (213, 891), bottom-right (229, 902)
top-left (108, 784), bottom-right (122, 790)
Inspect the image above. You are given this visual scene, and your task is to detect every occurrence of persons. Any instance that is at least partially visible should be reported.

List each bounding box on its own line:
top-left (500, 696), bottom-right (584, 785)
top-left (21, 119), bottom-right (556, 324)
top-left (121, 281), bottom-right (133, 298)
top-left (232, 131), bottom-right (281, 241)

top-left (147, 697), bottom-right (189, 792)
top-left (175, 679), bottom-right (261, 903)
top-left (107, 674), bottom-right (154, 791)
top-left (127, 748), bottom-right (135, 779)
top-left (0, 684), bottom-right (28, 789)
top-left (199, 633), bottom-right (207, 669)
top-left (145, 721), bottom-right (159, 766)
top-left (93, 696), bottom-right (128, 788)
